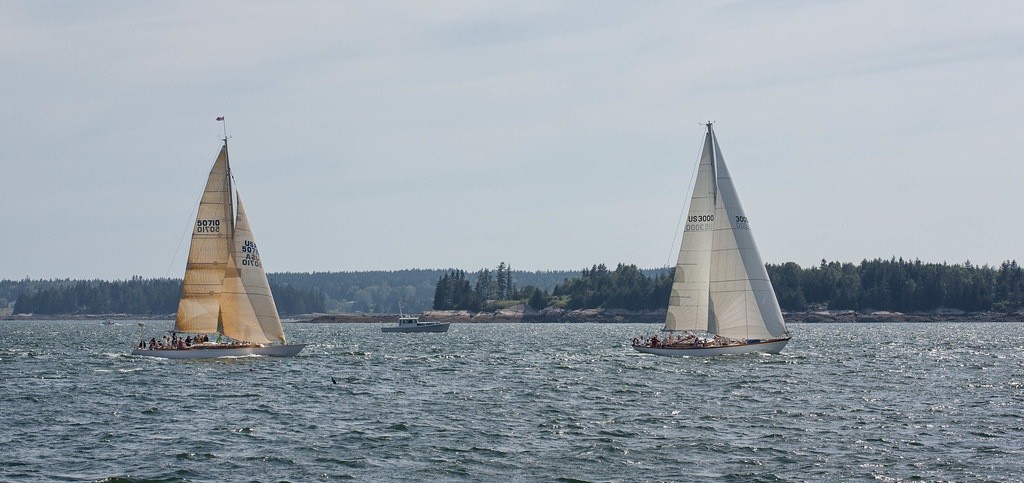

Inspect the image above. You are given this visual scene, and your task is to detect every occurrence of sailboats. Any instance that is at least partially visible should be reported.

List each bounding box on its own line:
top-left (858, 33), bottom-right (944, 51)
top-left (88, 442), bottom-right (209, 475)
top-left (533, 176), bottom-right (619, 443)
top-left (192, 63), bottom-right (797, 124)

top-left (132, 114), bottom-right (307, 361)
top-left (623, 117), bottom-right (794, 360)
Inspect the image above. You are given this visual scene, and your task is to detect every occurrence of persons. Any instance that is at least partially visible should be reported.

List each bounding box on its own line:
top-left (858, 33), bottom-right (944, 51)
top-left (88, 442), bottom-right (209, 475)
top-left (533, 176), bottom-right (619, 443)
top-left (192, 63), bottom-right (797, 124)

top-left (235, 341), bottom-right (251, 347)
top-left (639, 330), bottom-right (720, 348)
top-left (139, 333), bottom-right (209, 350)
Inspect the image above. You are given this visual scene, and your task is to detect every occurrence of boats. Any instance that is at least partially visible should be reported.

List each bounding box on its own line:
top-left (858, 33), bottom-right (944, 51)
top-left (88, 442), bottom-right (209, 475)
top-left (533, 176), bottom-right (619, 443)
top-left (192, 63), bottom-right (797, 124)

top-left (381, 317), bottom-right (449, 333)
